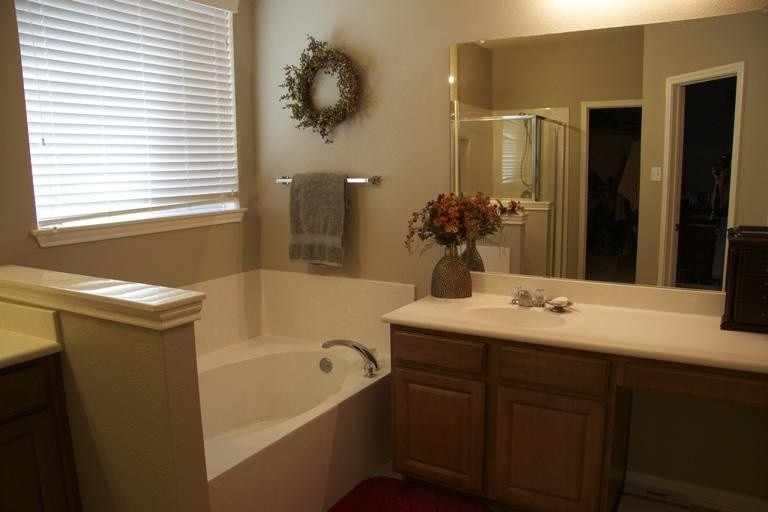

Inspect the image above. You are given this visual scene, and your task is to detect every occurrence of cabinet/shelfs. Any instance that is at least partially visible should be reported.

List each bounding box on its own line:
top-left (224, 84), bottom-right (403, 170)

top-left (390, 324), bottom-right (610, 512)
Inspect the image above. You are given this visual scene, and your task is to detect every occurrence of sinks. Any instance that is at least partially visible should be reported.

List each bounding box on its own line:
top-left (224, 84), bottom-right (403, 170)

top-left (449, 297), bottom-right (575, 356)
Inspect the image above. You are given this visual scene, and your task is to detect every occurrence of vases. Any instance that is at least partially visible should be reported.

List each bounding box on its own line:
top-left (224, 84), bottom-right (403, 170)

top-left (462, 241), bottom-right (485, 270)
top-left (431, 242), bottom-right (471, 298)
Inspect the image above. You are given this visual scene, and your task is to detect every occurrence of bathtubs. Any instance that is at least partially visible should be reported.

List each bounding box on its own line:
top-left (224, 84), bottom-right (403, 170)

top-left (195, 335), bottom-right (416, 512)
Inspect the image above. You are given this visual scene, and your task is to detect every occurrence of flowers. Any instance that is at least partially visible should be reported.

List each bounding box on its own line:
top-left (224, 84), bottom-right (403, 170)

top-left (458, 192), bottom-right (520, 241)
top-left (405, 184), bottom-right (478, 247)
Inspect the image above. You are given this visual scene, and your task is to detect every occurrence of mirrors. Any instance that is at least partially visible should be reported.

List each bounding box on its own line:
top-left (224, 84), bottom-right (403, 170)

top-left (449, 8), bottom-right (767, 294)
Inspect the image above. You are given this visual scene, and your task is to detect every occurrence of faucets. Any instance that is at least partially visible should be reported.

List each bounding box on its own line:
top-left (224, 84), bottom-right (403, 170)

top-left (515, 288), bottom-right (534, 307)
top-left (322, 339), bottom-right (380, 376)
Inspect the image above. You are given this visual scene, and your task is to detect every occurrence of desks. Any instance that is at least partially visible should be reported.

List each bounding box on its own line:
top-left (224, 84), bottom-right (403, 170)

top-left (0, 323), bottom-right (83, 512)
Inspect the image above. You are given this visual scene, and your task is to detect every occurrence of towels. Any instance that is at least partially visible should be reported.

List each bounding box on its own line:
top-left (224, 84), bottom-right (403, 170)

top-left (287, 172), bottom-right (345, 267)
